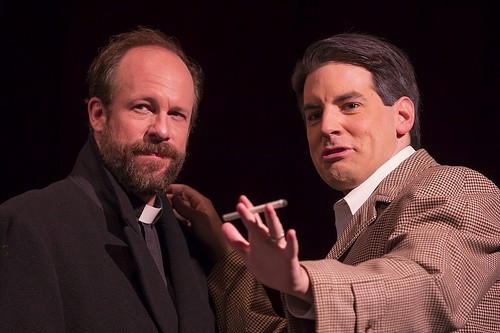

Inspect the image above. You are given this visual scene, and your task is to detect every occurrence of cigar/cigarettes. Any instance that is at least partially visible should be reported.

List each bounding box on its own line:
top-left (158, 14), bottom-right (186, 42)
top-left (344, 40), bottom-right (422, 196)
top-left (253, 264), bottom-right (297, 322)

top-left (223, 198), bottom-right (288, 221)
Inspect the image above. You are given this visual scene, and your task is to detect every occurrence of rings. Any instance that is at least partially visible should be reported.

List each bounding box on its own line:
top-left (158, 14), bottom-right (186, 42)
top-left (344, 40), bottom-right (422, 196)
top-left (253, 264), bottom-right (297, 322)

top-left (269, 233), bottom-right (285, 243)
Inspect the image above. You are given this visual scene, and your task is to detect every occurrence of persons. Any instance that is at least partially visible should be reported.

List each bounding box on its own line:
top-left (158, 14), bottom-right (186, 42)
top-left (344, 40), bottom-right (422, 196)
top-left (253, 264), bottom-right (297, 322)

top-left (162, 31), bottom-right (500, 333)
top-left (1, 28), bottom-right (219, 333)
